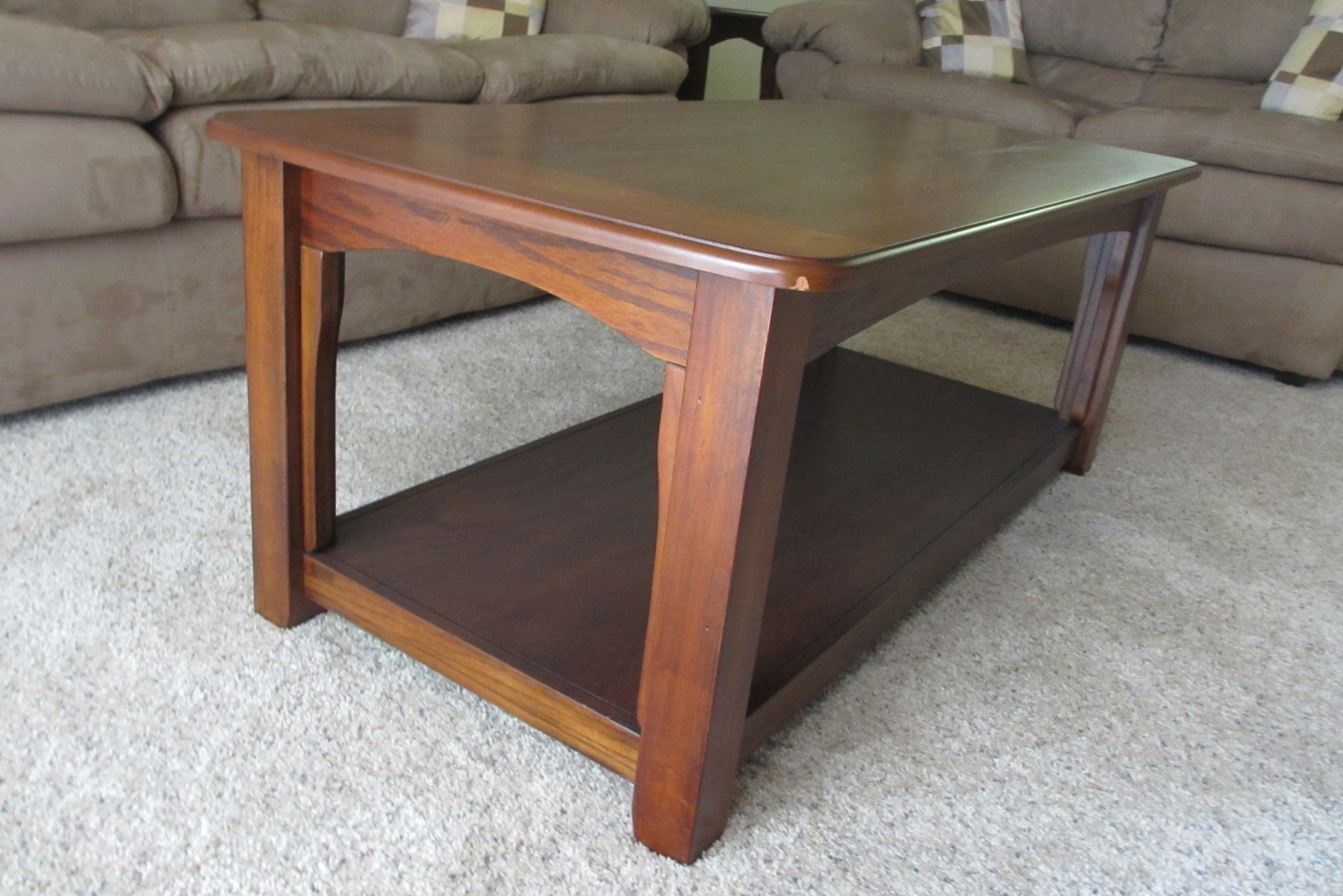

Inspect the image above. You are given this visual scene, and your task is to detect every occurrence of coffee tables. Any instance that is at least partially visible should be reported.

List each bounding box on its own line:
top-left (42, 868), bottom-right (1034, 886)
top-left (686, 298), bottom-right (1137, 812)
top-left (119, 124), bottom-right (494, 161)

top-left (204, 97), bottom-right (1202, 865)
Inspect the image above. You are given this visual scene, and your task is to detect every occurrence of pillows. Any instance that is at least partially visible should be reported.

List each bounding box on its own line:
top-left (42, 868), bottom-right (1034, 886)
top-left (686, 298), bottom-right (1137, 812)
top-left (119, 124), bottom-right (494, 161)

top-left (1257, 0), bottom-right (1343, 121)
top-left (406, 0), bottom-right (545, 46)
top-left (917, 0), bottom-right (1035, 88)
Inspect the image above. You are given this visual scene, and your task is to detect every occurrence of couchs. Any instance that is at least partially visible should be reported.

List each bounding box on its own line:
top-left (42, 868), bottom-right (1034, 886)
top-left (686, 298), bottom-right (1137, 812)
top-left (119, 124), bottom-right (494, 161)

top-left (0, 0), bottom-right (1342, 382)
